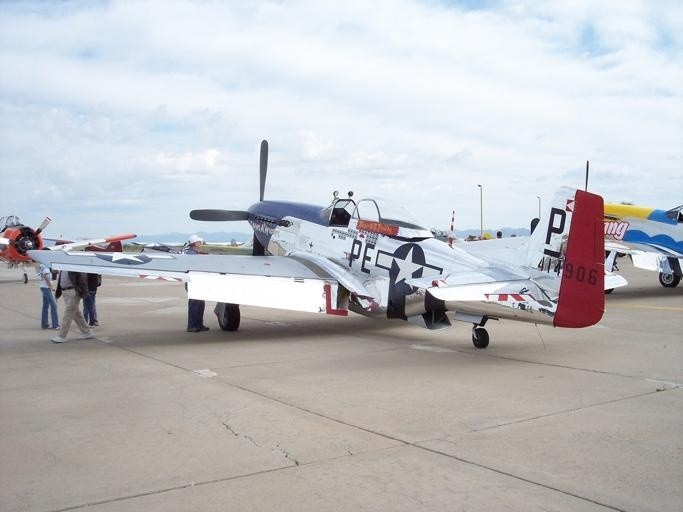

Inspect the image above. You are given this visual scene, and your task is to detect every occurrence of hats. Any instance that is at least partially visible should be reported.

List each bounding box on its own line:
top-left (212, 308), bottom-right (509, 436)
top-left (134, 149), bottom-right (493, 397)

top-left (188, 235), bottom-right (202, 245)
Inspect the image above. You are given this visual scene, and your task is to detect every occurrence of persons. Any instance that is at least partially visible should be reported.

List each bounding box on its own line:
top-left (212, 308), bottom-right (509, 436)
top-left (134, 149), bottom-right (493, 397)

top-left (183, 234), bottom-right (210, 333)
top-left (37, 246), bottom-right (62, 331)
top-left (50, 240), bottom-right (97, 344)
top-left (82, 272), bottom-right (103, 326)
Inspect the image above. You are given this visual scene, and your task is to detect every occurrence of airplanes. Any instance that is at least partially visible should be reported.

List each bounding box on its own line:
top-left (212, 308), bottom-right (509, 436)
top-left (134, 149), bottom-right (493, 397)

top-left (0, 213), bottom-right (141, 271)
top-left (27, 139), bottom-right (628, 349)
top-left (566, 191), bottom-right (683, 292)
top-left (129, 242), bottom-right (186, 255)
top-left (218, 239), bottom-right (247, 247)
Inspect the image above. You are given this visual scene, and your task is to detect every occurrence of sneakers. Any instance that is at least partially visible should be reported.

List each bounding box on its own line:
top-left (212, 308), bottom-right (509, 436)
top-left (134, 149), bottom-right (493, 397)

top-left (51, 337), bottom-right (67, 343)
top-left (78, 330), bottom-right (95, 340)
top-left (188, 325), bottom-right (209, 331)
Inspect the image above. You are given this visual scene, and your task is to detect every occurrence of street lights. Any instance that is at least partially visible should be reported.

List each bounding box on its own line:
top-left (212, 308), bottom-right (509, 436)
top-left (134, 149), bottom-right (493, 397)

top-left (537, 193), bottom-right (541, 221)
top-left (477, 184), bottom-right (483, 239)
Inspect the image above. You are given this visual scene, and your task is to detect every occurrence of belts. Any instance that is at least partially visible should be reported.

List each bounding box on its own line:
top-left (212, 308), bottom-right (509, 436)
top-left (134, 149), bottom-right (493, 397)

top-left (61, 286), bottom-right (74, 290)
